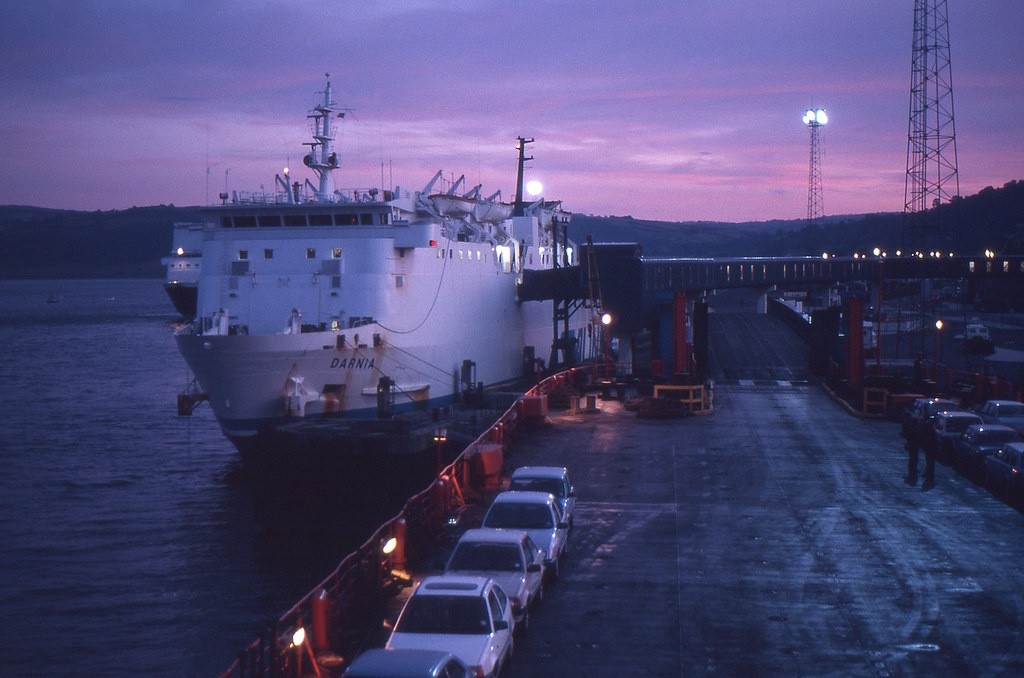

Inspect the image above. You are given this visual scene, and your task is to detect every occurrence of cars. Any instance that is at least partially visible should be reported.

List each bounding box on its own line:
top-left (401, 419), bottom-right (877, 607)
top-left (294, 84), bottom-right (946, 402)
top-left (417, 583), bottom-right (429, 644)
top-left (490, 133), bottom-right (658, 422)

top-left (970, 399), bottom-right (1024, 437)
top-left (382, 574), bottom-right (519, 678)
top-left (930, 412), bottom-right (985, 461)
top-left (983, 440), bottom-right (1024, 507)
top-left (908, 397), bottom-right (966, 442)
top-left (508, 465), bottom-right (576, 530)
top-left (479, 490), bottom-right (570, 578)
top-left (338, 648), bottom-right (478, 677)
top-left (442, 528), bottom-right (546, 632)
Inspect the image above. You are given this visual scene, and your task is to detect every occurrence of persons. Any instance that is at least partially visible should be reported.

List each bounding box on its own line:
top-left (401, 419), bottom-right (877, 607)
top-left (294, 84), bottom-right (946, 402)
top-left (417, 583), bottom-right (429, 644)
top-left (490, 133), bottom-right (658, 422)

top-left (900, 411), bottom-right (939, 484)
top-left (913, 355), bottom-right (926, 386)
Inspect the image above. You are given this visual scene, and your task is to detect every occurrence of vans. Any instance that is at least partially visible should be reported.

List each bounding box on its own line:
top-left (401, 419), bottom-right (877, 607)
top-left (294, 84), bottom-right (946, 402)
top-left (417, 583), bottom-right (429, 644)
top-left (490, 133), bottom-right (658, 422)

top-left (958, 423), bottom-right (1022, 476)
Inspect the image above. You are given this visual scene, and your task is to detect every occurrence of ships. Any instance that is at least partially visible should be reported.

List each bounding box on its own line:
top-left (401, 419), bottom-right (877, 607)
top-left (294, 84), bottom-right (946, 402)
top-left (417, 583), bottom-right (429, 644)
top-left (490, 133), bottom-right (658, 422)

top-left (162, 69), bottom-right (599, 453)
